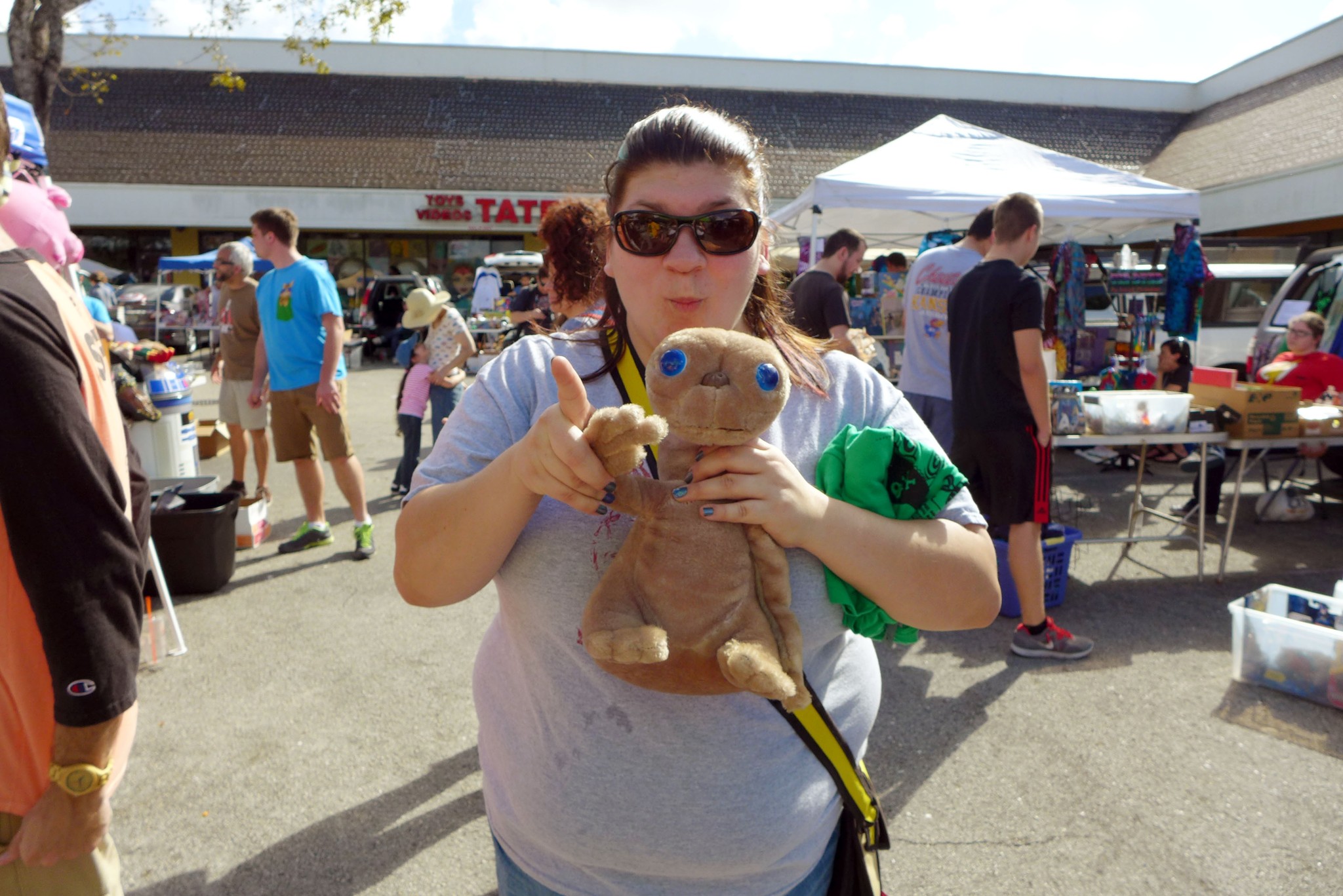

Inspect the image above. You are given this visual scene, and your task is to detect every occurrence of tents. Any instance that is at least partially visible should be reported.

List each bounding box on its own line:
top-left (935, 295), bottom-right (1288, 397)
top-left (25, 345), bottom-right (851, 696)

top-left (760, 113), bottom-right (1204, 365)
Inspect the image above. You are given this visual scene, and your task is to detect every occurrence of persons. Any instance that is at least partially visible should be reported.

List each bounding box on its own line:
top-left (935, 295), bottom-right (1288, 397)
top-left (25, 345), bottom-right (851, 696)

top-left (781, 227), bottom-right (869, 363)
top-left (948, 191), bottom-right (1097, 659)
top-left (392, 106), bottom-right (1003, 896)
top-left (1146, 339), bottom-right (1197, 463)
top-left (1170, 308), bottom-right (1343, 518)
top-left (887, 251), bottom-right (907, 272)
top-left (0, 84), bottom-right (150, 896)
top-left (899, 204), bottom-right (999, 462)
top-left (77, 198), bottom-right (611, 561)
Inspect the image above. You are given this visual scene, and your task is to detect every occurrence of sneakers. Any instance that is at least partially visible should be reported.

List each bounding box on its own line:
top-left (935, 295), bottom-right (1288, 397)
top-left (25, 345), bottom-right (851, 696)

top-left (396, 483), bottom-right (409, 498)
top-left (276, 520), bottom-right (334, 554)
top-left (217, 483), bottom-right (249, 496)
top-left (351, 523), bottom-right (377, 561)
top-left (389, 479), bottom-right (400, 495)
top-left (1009, 618), bottom-right (1094, 661)
top-left (254, 487), bottom-right (274, 509)
top-left (1169, 498), bottom-right (1216, 520)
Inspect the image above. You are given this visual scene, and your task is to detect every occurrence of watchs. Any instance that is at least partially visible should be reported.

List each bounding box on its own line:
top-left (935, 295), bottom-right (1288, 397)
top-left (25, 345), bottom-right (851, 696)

top-left (48, 758), bottom-right (112, 796)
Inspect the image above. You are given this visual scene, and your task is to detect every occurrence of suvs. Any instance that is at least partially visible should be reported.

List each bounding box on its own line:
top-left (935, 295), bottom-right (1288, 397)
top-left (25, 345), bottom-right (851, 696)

top-left (352, 270), bottom-right (449, 362)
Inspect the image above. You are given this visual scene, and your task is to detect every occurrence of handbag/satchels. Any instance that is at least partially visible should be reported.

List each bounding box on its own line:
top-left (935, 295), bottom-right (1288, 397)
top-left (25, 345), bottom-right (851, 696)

top-left (830, 762), bottom-right (898, 896)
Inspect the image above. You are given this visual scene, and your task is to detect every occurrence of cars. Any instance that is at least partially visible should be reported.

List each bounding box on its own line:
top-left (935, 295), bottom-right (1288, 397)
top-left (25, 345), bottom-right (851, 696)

top-left (1023, 244), bottom-right (1342, 480)
top-left (113, 280), bottom-right (218, 354)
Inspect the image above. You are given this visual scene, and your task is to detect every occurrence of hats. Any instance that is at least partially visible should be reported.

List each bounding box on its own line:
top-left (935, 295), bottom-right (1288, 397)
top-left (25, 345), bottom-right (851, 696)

top-left (400, 287), bottom-right (452, 328)
top-left (395, 330), bottom-right (426, 370)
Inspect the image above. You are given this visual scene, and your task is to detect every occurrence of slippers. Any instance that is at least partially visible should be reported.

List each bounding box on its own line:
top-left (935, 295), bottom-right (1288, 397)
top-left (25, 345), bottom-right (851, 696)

top-left (1155, 449), bottom-right (1190, 464)
top-left (1144, 447), bottom-right (1170, 460)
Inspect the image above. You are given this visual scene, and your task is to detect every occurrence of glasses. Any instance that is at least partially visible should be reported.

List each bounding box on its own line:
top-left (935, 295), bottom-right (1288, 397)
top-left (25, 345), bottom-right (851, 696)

top-left (1286, 327), bottom-right (1319, 338)
top-left (538, 276), bottom-right (550, 287)
top-left (607, 204), bottom-right (764, 258)
top-left (212, 257), bottom-right (236, 267)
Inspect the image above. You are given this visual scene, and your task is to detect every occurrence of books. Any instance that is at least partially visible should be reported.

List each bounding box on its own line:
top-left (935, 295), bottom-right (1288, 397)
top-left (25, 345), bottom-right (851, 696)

top-left (1192, 366), bottom-right (1238, 389)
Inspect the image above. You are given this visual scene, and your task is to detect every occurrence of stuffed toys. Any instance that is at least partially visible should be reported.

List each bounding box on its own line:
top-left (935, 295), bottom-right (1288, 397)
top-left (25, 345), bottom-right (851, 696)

top-left (575, 328), bottom-right (812, 711)
top-left (0, 177), bottom-right (84, 273)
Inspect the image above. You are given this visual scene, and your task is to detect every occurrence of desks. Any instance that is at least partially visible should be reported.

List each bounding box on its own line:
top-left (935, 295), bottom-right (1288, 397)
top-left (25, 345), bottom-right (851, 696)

top-left (460, 328), bottom-right (512, 374)
top-left (1050, 430), bottom-right (1229, 584)
top-left (1163, 434), bottom-right (1343, 584)
top-left (868, 334), bottom-right (905, 387)
top-left (158, 322), bottom-right (221, 363)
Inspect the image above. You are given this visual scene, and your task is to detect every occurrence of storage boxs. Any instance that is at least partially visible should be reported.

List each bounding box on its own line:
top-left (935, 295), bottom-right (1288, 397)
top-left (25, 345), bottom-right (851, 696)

top-left (195, 416), bottom-right (231, 460)
top-left (1048, 366), bottom-right (1343, 441)
top-left (843, 268), bottom-right (908, 336)
top-left (144, 476), bottom-right (273, 598)
top-left (342, 337), bottom-right (368, 371)
top-left (987, 519), bottom-right (1082, 618)
top-left (478, 295), bottom-right (512, 328)
top-left (1226, 582), bottom-right (1342, 711)
